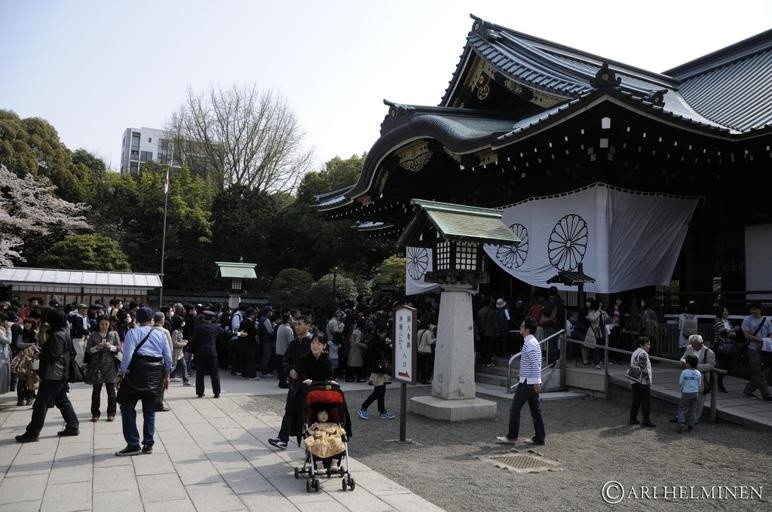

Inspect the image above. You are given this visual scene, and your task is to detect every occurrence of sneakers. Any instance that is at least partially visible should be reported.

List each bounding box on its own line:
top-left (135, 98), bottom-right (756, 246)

top-left (742, 389), bottom-right (759, 401)
top-left (317, 465), bottom-right (325, 474)
top-left (763, 392), bottom-right (772, 400)
top-left (142, 444), bottom-right (153, 454)
top-left (162, 404), bottom-right (169, 410)
top-left (526, 438), bottom-right (536, 443)
top-left (676, 424), bottom-right (684, 432)
top-left (114, 446), bottom-right (141, 456)
top-left (379, 411), bottom-right (396, 419)
top-left (688, 423), bottom-right (693, 428)
top-left (329, 463), bottom-right (338, 471)
top-left (268, 438), bottom-right (287, 449)
top-left (357, 407), bottom-right (369, 420)
top-left (496, 436), bottom-right (517, 444)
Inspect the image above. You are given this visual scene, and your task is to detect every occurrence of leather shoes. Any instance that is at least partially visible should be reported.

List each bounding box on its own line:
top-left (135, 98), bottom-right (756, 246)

top-left (642, 419), bottom-right (656, 427)
top-left (718, 381), bottom-right (728, 393)
top-left (58, 427), bottom-right (79, 436)
top-left (670, 417), bottom-right (678, 422)
top-left (108, 416), bottom-right (114, 421)
top-left (630, 419), bottom-right (640, 424)
top-left (15, 435), bottom-right (38, 442)
top-left (92, 416), bottom-right (98, 421)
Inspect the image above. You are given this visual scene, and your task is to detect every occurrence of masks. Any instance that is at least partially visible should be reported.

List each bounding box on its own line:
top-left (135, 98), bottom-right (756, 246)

top-left (180, 321), bottom-right (186, 328)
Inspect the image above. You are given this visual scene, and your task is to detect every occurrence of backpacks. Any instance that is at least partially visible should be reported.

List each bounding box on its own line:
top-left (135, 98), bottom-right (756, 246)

top-left (69, 359), bottom-right (84, 383)
top-left (684, 313), bottom-right (697, 338)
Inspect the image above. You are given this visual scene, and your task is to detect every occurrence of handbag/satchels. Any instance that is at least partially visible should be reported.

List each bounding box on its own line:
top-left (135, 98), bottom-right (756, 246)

top-left (419, 329), bottom-right (427, 346)
top-left (68, 360), bottom-right (85, 382)
top-left (127, 327), bottom-right (155, 370)
top-left (626, 350), bottom-right (644, 383)
top-left (703, 349), bottom-right (713, 394)
top-left (742, 318), bottom-right (766, 361)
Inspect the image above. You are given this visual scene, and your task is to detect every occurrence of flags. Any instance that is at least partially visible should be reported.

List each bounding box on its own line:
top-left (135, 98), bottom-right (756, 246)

top-left (163, 169), bottom-right (170, 193)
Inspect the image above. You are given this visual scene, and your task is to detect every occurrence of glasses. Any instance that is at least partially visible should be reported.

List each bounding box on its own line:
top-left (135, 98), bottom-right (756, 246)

top-left (518, 325), bottom-right (525, 328)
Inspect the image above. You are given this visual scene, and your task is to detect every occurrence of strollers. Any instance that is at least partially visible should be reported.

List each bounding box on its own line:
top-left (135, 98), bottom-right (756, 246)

top-left (295, 381), bottom-right (354, 493)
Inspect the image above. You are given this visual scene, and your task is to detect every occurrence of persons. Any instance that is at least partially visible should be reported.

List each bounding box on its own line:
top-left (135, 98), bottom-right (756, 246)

top-left (675, 355), bottom-right (702, 432)
top-left (417, 318), bottom-right (439, 384)
top-left (84, 315), bottom-right (121, 421)
top-left (154, 302), bottom-right (199, 337)
top-left (741, 301), bottom-right (772, 404)
top-left (298, 332), bottom-right (338, 385)
top-left (345, 319), bottom-right (367, 383)
top-left (629, 337), bottom-right (657, 427)
top-left (0, 311), bottom-right (12, 395)
top-left (679, 299), bottom-right (698, 357)
top-left (308, 408), bottom-right (343, 475)
top-left (578, 297), bottom-right (594, 366)
top-left (16, 320), bottom-right (34, 407)
top-left (584, 299), bottom-right (610, 369)
top-left (712, 303), bottom-right (739, 393)
top-left (477, 297), bottom-right (499, 368)
top-left (238, 307), bottom-right (261, 381)
top-left (566, 312), bottom-right (575, 337)
top-left (606, 296), bottom-right (626, 365)
top-left (496, 298), bottom-right (510, 358)
top-left (639, 297), bottom-right (660, 364)
top-left (357, 320), bottom-right (396, 419)
top-left (540, 286), bottom-right (565, 369)
top-left (91, 302), bottom-right (105, 332)
top-left (326, 311), bottom-right (345, 385)
top-left (528, 297), bottom-right (545, 357)
top-left (276, 313), bottom-right (294, 388)
top-left (65, 303), bottom-right (90, 368)
top-left (115, 306), bottom-right (174, 457)
top-left (106, 299), bottom-right (139, 342)
top-left (259, 306), bottom-right (275, 378)
top-left (185, 311), bottom-right (247, 398)
top-left (199, 303), bottom-right (230, 330)
top-left (669, 335), bottom-right (716, 433)
top-left (268, 315), bottom-right (314, 450)
top-left (0, 297), bottom-right (38, 356)
top-left (172, 315), bottom-right (195, 387)
top-left (496, 319), bottom-right (545, 444)
top-left (231, 302), bottom-right (249, 376)
top-left (14, 308), bottom-right (79, 443)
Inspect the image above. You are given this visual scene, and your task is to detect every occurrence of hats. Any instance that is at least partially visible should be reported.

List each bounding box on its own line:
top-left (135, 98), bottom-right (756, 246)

top-left (496, 298), bottom-right (506, 308)
top-left (263, 306), bottom-right (274, 312)
top-left (137, 306), bottom-right (154, 318)
top-left (79, 304), bottom-right (88, 309)
top-left (203, 310), bottom-right (216, 319)
top-left (245, 307), bottom-right (257, 316)
